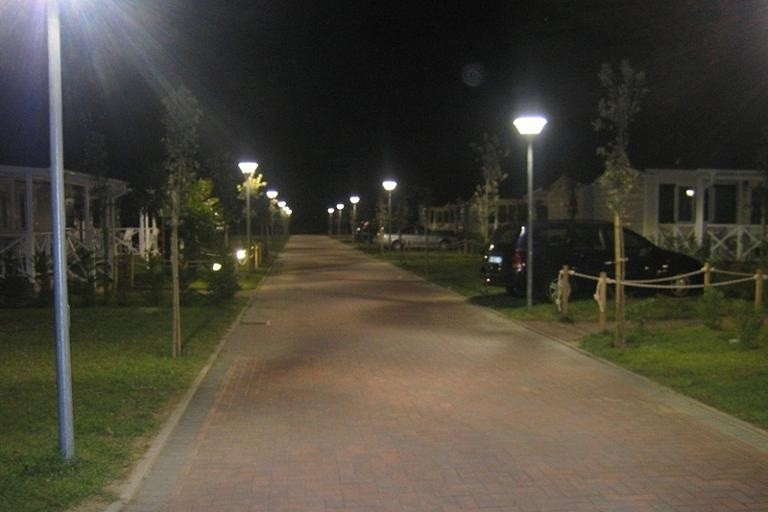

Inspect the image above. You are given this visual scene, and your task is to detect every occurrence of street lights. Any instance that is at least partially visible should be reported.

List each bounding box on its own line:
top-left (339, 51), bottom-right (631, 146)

top-left (381, 180), bottom-right (397, 255)
top-left (513, 115), bottom-right (547, 310)
top-left (349, 195), bottom-right (360, 248)
top-left (267, 190), bottom-right (292, 261)
top-left (336, 203), bottom-right (344, 239)
top-left (327, 207), bottom-right (334, 237)
top-left (237, 160), bottom-right (259, 276)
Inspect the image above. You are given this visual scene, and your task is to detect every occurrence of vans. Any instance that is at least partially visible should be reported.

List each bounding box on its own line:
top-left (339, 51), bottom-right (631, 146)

top-left (358, 220), bottom-right (380, 241)
top-left (377, 224), bottom-right (460, 250)
top-left (482, 217), bottom-right (704, 300)
